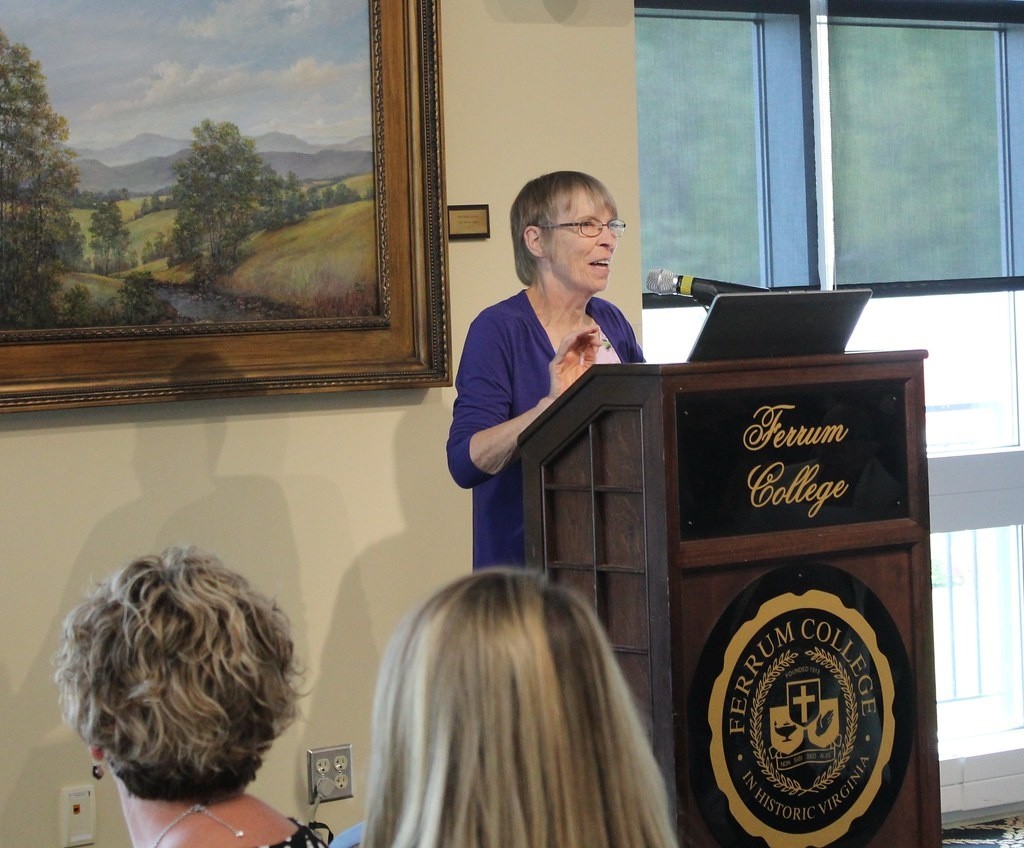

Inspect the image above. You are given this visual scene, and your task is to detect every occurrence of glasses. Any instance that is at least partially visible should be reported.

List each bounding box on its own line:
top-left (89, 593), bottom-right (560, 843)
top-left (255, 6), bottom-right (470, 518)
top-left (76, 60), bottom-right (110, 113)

top-left (535, 218), bottom-right (627, 238)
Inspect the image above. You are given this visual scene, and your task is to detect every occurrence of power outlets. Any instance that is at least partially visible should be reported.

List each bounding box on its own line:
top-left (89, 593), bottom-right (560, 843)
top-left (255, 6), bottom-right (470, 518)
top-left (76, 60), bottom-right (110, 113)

top-left (306, 744), bottom-right (353, 805)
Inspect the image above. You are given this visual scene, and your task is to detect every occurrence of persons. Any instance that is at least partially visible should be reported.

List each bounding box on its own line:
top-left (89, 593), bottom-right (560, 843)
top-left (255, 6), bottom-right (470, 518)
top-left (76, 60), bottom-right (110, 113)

top-left (447, 171), bottom-right (651, 571)
top-left (329, 569), bottom-right (678, 848)
top-left (54, 546), bottom-right (333, 848)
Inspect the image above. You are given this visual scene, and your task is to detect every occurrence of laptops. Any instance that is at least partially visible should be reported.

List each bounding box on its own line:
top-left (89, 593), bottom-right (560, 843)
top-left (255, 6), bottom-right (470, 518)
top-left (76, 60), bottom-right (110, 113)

top-left (687, 289), bottom-right (873, 363)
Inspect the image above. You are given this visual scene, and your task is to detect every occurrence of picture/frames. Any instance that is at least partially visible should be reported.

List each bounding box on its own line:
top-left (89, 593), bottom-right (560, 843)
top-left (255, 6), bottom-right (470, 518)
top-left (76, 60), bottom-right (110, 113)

top-left (0, 0), bottom-right (453, 413)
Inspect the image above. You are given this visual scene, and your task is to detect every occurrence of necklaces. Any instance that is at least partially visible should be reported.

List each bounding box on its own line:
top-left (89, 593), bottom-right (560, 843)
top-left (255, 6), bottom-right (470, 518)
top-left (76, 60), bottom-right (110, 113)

top-left (154, 802), bottom-right (244, 848)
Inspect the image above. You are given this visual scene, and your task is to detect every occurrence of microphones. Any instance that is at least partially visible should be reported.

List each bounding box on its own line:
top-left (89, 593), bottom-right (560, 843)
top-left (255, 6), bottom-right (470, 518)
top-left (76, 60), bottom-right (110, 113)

top-left (646, 269), bottom-right (771, 305)
